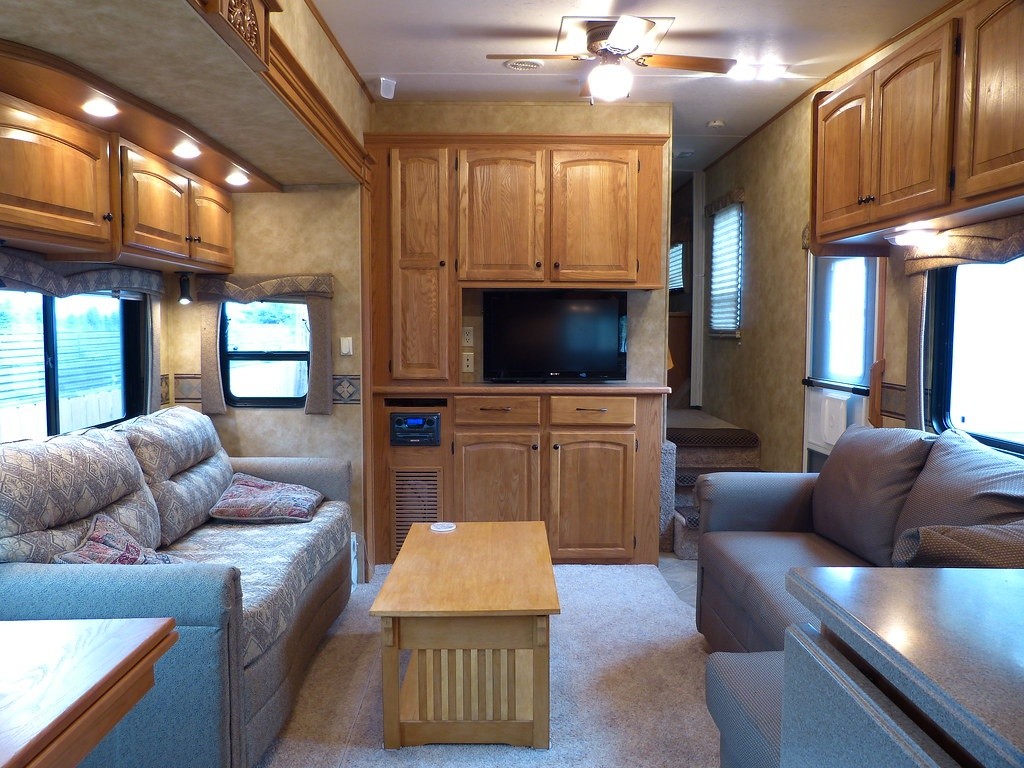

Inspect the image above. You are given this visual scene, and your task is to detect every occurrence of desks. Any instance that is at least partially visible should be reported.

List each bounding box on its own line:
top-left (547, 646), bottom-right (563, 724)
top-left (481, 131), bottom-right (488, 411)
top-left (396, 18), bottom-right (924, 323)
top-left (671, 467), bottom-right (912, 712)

top-left (0, 617), bottom-right (179, 768)
top-left (780, 566), bottom-right (1024, 768)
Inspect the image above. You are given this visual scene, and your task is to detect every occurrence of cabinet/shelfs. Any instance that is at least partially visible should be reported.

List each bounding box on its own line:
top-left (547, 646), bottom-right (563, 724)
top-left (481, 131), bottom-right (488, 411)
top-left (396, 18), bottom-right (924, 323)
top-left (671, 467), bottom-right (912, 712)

top-left (0, 91), bottom-right (111, 255)
top-left (952, 1), bottom-right (1024, 223)
top-left (810, 17), bottom-right (951, 257)
top-left (119, 134), bottom-right (235, 274)
top-left (362, 133), bottom-right (670, 566)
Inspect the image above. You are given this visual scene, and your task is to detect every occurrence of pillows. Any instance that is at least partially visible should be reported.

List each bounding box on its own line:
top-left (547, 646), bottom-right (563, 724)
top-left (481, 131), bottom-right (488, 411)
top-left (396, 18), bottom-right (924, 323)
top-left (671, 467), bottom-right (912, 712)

top-left (54, 513), bottom-right (193, 565)
top-left (210, 472), bottom-right (323, 524)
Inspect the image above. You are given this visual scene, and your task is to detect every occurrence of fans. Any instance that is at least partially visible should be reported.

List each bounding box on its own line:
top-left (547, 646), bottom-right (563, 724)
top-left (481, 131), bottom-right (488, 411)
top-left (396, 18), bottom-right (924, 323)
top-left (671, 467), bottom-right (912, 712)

top-left (486, 14), bottom-right (737, 98)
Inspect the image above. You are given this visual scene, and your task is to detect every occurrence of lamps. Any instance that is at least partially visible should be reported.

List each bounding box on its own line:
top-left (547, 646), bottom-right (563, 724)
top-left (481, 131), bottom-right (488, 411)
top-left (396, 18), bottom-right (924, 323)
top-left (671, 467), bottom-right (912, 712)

top-left (587, 55), bottom-right (635, 106)
top-left (178, 274), bottom-right (193, 306)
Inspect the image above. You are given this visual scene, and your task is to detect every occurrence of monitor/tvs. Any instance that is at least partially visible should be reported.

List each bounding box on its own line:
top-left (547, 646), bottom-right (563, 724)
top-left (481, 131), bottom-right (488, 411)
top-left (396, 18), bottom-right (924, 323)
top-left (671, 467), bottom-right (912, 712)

top-left (483, 290), bottom-right (627, 384)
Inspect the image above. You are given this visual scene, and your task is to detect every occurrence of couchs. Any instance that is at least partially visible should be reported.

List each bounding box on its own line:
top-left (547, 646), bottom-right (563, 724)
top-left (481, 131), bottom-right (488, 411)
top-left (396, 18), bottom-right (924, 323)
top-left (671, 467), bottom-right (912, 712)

top-left (695, 423), bottom-right (1024, 768)
top-left (0, 404), bottom-right (353, 768)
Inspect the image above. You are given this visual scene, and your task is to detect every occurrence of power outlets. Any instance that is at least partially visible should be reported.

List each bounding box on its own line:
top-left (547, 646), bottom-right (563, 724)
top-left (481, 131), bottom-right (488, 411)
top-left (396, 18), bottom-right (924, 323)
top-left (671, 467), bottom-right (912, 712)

top-left (462, 327), bottom-right (474, 347)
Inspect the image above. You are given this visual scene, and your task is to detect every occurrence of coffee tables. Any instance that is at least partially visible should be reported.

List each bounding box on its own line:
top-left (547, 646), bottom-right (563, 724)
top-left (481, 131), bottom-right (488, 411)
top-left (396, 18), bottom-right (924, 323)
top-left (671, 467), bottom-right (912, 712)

top-left (370, 521), bottom-right (560, 751)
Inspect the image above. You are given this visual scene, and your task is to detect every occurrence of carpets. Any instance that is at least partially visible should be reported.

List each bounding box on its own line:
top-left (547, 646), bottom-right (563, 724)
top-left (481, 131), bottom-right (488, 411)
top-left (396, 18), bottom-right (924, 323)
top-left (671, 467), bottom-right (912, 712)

top-left (262, 564), bottom-right (721, 768)
